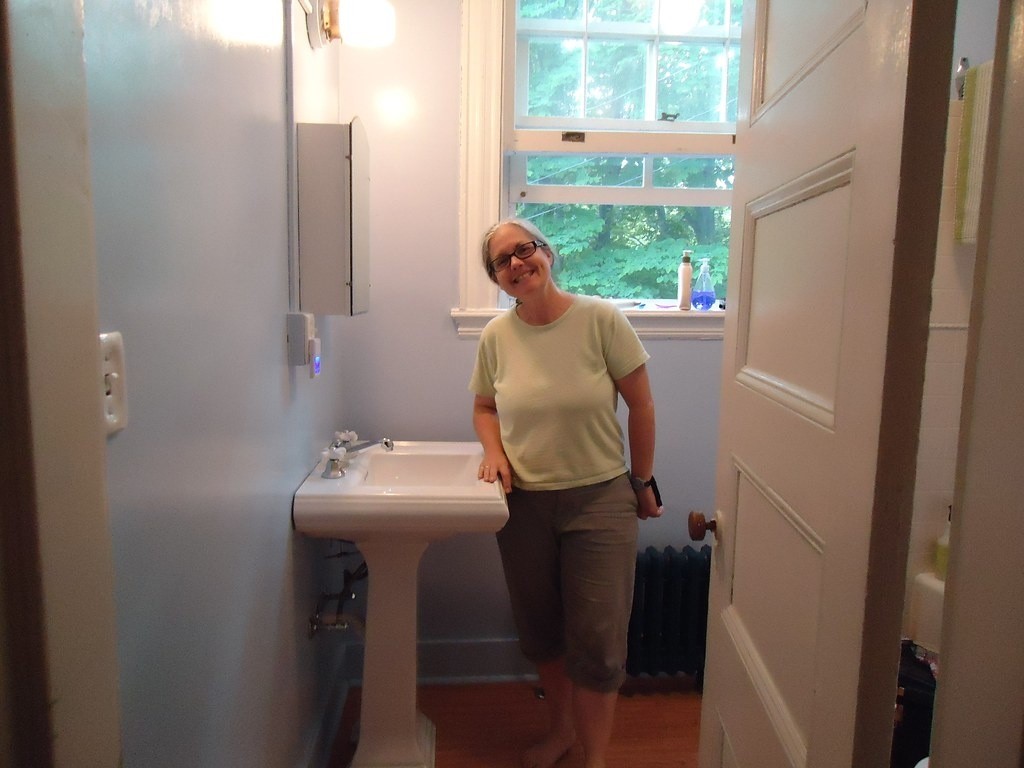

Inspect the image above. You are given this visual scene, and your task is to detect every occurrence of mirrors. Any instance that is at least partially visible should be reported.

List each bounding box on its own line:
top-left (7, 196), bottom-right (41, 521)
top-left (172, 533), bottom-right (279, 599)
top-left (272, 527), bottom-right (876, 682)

top-left (348, 116), bottom-right (374, 317)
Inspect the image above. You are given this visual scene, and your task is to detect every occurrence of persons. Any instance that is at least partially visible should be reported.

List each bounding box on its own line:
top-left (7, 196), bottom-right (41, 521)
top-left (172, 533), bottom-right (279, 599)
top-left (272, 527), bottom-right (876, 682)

top-left (467, 217), bottom-right (665, 768)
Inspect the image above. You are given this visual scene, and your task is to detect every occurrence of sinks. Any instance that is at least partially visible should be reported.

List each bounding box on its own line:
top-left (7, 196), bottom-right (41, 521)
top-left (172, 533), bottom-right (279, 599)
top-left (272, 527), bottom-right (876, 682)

top-left (293, 440), bottom-right (510, 539)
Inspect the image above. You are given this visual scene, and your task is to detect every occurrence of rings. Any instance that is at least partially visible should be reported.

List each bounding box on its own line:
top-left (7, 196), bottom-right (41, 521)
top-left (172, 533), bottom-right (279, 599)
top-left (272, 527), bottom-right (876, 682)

top-left (485, 466), bottom-right (490, 469)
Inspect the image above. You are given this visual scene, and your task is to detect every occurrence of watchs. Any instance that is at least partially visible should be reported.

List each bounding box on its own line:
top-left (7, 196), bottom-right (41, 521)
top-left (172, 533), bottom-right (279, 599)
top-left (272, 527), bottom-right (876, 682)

top-left (630, 476), bottom-right (652, 491)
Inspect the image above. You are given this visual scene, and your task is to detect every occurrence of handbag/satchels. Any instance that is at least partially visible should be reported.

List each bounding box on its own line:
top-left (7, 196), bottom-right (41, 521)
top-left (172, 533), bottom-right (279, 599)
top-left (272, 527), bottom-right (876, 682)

top-left (890, 639), bottom-right (936, 768)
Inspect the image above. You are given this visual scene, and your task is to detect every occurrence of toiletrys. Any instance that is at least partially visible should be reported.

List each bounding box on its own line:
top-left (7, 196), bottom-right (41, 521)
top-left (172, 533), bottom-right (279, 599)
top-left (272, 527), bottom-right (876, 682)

top-left (677, 250), bottom-right (716, 311)
top-left (934, 505), bottom-right (954, 583)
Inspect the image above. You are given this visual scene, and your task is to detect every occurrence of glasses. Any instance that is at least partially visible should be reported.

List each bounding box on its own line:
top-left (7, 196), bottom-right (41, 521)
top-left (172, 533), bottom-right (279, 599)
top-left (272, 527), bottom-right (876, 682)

top-left (488, 241), bottom-right (547, 272)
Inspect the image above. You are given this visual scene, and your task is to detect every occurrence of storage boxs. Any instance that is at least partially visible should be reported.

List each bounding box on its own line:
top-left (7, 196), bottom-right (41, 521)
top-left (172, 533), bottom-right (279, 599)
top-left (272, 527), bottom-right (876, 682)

top-left (536, 544), bottom-right (712, 699)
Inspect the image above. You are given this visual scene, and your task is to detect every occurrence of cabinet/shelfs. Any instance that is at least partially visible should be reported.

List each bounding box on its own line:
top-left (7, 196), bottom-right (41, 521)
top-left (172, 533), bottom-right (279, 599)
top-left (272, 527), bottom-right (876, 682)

top-left (297, 116), bottom-right (372, 317)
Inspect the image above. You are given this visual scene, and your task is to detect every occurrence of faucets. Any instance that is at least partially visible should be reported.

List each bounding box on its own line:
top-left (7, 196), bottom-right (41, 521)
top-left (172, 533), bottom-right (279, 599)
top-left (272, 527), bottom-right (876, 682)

top-left (329, 437), bottom-right (394, 469)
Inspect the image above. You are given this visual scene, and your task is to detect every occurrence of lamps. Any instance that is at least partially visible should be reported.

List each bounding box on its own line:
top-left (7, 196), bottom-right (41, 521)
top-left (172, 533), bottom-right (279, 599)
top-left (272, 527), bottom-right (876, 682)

top-left (306, 0), bottom-right (396, 53)
